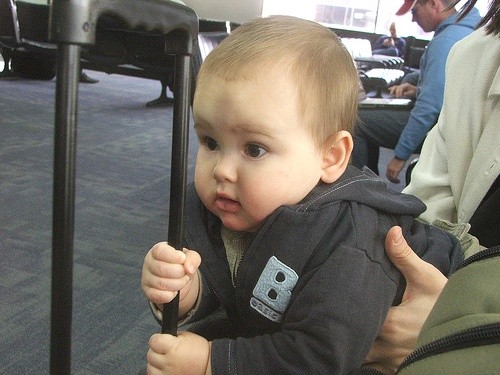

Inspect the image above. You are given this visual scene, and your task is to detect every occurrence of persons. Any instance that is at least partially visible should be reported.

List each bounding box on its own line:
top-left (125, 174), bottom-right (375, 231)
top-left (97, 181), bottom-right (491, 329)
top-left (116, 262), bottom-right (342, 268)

top-left (355, 0), bottom-right (500, 375)
top-left (140, 15), bottom-right (489, 375)
top-left (347, 0), bottom-right (486, 184)
top-left (371, 22), bottom-right (407, 59)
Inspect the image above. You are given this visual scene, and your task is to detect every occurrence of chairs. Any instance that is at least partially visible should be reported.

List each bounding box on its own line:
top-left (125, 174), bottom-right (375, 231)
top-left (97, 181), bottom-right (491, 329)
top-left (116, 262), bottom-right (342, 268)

top-left (0, 0), bottom-right (432, 110)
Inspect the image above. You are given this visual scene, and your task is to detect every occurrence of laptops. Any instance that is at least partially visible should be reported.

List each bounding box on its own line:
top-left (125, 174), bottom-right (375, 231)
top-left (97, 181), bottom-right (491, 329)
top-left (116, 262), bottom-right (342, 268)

top-left (357, 76), bottom-right (414, 109)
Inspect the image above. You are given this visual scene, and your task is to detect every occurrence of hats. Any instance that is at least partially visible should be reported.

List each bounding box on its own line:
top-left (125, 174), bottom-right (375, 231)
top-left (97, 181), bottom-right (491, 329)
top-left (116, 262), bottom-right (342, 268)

top-left (395, 0), bottom-right (414, 15)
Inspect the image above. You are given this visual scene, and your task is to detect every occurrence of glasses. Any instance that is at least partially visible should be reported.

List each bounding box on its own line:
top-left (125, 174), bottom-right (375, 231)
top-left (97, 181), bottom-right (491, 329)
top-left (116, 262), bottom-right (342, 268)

top-left (411, 4), bottom-right (422, 14)
